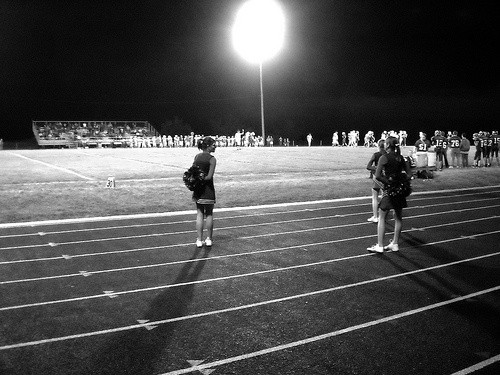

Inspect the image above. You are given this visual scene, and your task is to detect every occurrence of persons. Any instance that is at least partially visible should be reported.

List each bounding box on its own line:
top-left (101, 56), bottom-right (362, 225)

top-left (414, 130), bottom-right (500, 170)
top-left (332, 130), bottom-right (360, 147)
top-left (364, 131), bottom-right (375, 148)
top-left (366, 136), bottom-right (412, 253)
top-left (193, 137), bottom-right (217, 247)
top-left (38, 120), bottom-right (290, 149)
top-left (306, 133), bottom-right (313, 146)
top-left (381, 130), bottom-right (408, 146)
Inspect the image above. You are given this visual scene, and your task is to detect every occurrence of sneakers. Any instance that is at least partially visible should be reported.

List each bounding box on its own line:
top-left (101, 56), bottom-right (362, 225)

top-left (367, 243), bottom-right (383, 253)
top-left (384, 239), bottom-right (399, 252)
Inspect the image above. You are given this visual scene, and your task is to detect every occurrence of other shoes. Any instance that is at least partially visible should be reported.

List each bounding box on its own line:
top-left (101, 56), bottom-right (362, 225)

top-left (205, 237), bottom-right (212, 246)
top-left (196, 240), bottom-right (202, 248)
top-left (367, 215), bottom-right (378, 222)
top-left (436, 163), bottom-right (500, 171)
